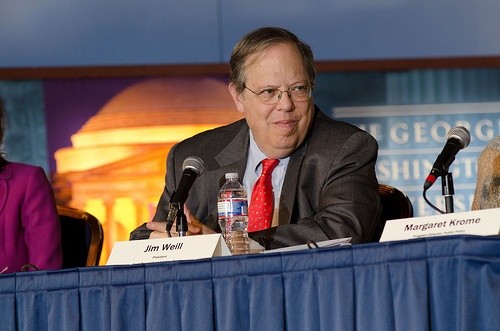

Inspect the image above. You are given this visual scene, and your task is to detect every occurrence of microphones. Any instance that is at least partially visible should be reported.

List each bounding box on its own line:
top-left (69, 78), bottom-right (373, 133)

top-left (424, 127), bottom-right (471, 190)
top-left (165, 155), bottom-right (204, 230)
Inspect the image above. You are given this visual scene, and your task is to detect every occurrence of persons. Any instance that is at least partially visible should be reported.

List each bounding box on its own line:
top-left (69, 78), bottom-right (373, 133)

top-left (129, 27), bottom-right (383, 250)
top-left (0, 95), bottom-right (62, 275)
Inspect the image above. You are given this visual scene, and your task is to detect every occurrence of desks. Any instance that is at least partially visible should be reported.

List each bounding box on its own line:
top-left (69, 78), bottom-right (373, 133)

top-left (0, 234), bottom-right (500, 331)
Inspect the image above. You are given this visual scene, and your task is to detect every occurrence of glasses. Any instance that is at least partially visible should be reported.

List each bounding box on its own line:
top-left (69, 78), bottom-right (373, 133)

top-left (243, 82), bottom-right (316, 106)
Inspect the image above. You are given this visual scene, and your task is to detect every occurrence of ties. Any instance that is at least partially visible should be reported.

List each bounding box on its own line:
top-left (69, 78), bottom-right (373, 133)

top-left (247, 159), bottom-right (280, 232)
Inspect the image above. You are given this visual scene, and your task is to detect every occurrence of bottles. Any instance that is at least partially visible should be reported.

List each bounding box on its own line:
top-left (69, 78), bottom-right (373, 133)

top-left (217, 173), bottom-right (250, 257)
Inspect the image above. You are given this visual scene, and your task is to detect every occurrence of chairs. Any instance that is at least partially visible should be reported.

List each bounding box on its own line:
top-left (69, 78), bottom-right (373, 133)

top-left (57, 205), bottom-right (104, 270)
top-left (369, 184), bottom-right (413, 243)
